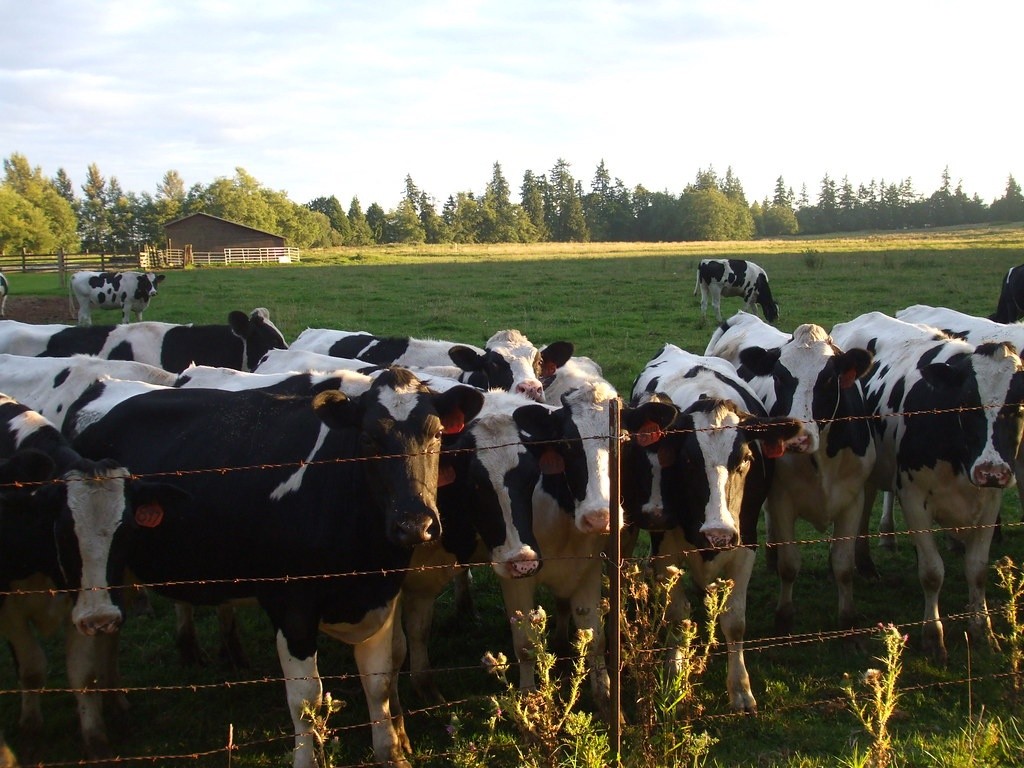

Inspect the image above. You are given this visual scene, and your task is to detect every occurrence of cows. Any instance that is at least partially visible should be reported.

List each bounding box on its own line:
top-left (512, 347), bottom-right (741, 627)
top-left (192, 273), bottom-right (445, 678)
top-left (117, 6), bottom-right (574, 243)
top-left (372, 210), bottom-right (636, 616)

top-left (0, 262), bottom-right (1024, 768)
top-left (693, 257), bottom-right (781, 324)
top-left (72, 269), bottom-right (164, 324)
